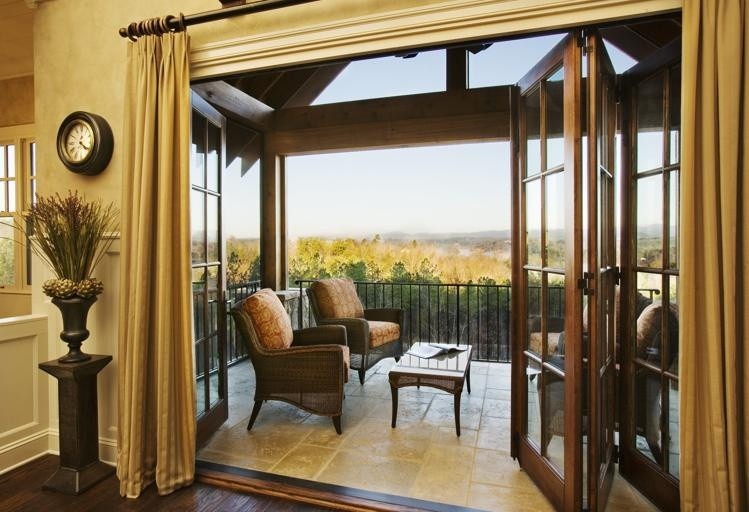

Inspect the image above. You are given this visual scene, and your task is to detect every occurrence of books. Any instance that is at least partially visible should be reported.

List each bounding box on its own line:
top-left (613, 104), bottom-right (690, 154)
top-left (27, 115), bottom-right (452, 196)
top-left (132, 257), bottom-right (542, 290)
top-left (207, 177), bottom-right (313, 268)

top-left (407, 342), bottom-right (468, 359)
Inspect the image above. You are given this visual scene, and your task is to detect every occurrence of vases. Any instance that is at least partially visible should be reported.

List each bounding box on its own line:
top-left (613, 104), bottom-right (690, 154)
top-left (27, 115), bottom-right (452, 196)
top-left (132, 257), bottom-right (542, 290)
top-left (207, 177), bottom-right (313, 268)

top-left (52, 296), bottom-right (98, 363)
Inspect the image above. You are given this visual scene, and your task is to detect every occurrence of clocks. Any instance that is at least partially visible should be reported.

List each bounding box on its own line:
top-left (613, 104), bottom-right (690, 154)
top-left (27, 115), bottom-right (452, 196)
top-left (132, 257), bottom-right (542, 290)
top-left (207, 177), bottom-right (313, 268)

top-left (56, 111), bottom-right (114, 176)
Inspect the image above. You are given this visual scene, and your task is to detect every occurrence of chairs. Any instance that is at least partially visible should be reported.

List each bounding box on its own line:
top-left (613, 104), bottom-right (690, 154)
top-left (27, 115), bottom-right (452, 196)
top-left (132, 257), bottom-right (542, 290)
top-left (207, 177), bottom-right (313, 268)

top-left (227, 277), bottom-right (404, 436)
top-left (523, 285), bottom-right (679, 470)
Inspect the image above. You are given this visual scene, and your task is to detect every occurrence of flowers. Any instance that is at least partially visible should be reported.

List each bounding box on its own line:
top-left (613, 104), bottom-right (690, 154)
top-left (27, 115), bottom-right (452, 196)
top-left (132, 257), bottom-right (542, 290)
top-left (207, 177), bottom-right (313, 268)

top-left (1, 189), bottom-right (122, 299)
